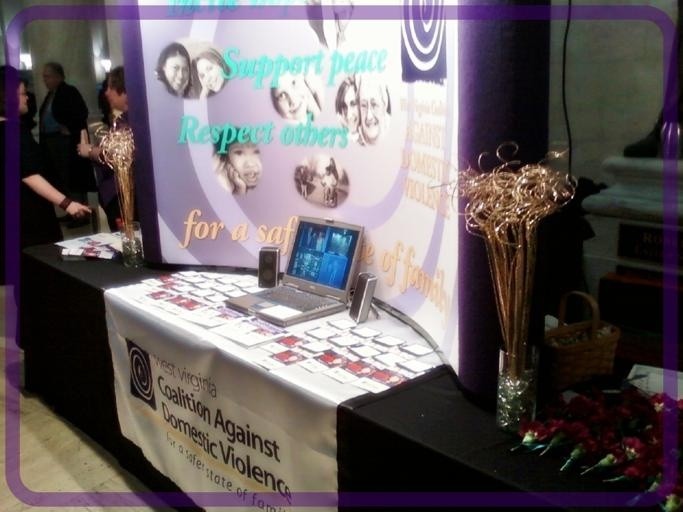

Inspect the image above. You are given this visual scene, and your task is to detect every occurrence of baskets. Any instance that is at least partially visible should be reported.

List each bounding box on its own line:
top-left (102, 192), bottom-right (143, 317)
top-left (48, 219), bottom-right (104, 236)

top-left (544, 289), bottom-right (621, 388)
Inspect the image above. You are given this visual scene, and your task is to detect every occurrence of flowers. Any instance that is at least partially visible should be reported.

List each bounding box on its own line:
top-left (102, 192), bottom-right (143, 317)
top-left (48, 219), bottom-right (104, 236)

top-left (510, 388), bottom-right (683, 512)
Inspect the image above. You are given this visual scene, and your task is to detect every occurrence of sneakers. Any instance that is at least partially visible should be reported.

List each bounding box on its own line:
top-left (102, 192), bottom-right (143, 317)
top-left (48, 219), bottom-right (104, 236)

top-left (56, 213), bottom-right (91, 230)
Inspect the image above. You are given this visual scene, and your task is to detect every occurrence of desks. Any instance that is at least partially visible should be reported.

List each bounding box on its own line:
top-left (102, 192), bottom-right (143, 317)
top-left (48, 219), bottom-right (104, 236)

top-left (18, 228), bottom-right (509, 510)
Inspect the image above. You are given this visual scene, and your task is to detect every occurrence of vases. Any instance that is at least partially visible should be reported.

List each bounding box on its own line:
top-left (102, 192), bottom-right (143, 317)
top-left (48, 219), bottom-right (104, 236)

top-left (121, 222), bottom-right (144, 269)
top-left (495, 345), bottom-right (537, 437)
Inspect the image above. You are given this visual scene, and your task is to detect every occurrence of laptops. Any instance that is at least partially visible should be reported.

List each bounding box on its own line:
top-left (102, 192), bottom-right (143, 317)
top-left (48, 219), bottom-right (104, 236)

top-left (225, 216), bottom-right (364, 327)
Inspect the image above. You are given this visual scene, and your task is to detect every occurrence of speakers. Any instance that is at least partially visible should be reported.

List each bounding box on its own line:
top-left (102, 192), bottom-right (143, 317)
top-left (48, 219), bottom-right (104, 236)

top-left (349, 272), bottom-right (377, 324)
top-left (258, 247), bottom-right (280, 288)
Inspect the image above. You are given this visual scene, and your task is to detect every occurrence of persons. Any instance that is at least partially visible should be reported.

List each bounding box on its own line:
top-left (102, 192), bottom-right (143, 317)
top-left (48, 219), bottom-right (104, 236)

top-left (320, 164), bottom-right (338, 203)
top-left (268, 71), bottom-right (319, 121)
top-left (189, 46), bottom-right (230, 99)
top-left (354, 72), bottom-right (392, 147)
top-left (291, 223), bottom-right (351, 287)
top-left (158, 43), bottom-right (192, 98)
top-left (209, 128), bottom-right (263, 196)
top-left (294, 163), bottom-right (308, 199)
top-left (1, 59), bottom-right (138, 351)
top-left (335, 73), bottom-right (363, 144)
top-left (328, 156), bottom-right (340, 202)
